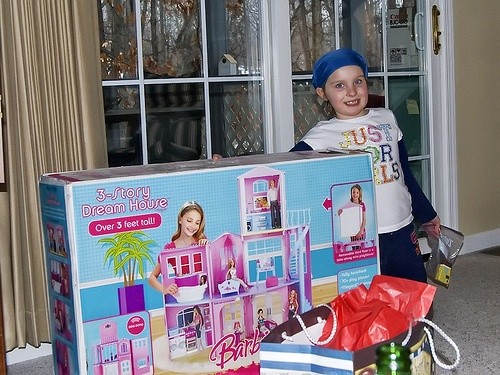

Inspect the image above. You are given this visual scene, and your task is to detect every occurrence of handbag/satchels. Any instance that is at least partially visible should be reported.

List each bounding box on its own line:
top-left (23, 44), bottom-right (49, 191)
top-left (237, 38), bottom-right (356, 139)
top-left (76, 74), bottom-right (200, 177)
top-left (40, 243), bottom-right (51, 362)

top-left (259, 298), bottom-right (461, 375)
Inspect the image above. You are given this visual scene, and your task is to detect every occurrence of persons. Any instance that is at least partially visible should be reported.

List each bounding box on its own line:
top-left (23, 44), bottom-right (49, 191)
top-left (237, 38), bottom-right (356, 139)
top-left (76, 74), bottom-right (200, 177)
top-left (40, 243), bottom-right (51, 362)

top-left (338, 184), bottom-right (366, 250)
top-left (213, 47), bottom-right (441, 283)
top-left (148, 201), bottom-right (209, 295)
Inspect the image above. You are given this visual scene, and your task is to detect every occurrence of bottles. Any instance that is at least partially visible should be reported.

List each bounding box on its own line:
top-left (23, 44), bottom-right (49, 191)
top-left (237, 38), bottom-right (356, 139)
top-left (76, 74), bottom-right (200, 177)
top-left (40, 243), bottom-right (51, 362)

top-left (375, 343), bottom-right (413, 375)
